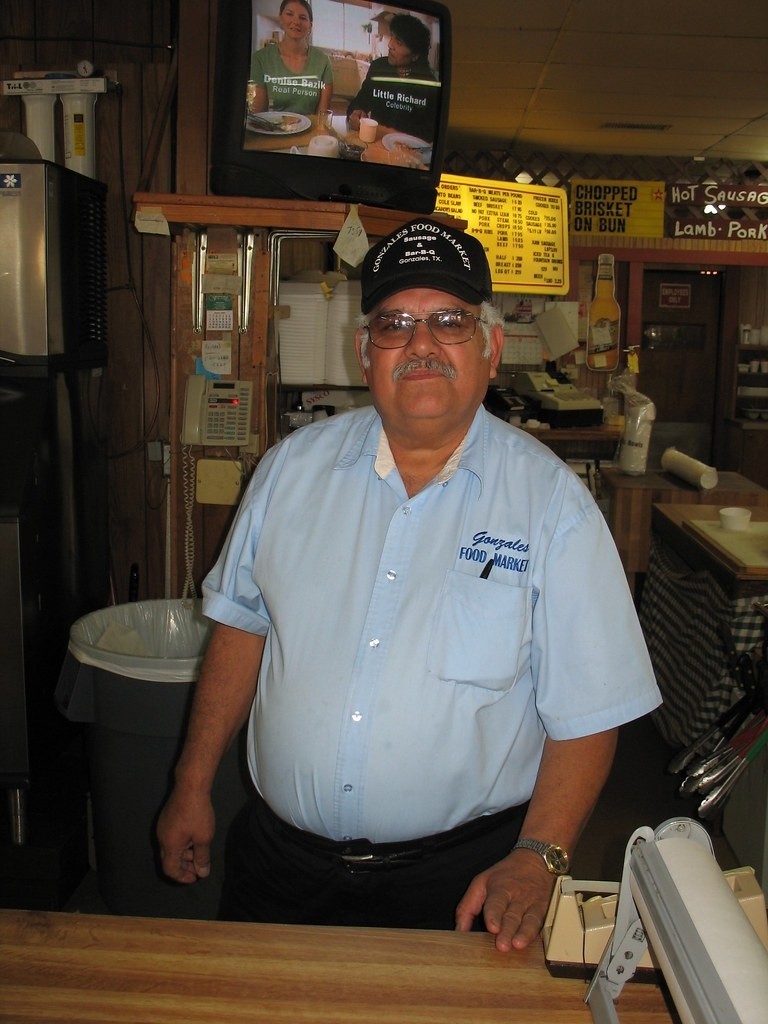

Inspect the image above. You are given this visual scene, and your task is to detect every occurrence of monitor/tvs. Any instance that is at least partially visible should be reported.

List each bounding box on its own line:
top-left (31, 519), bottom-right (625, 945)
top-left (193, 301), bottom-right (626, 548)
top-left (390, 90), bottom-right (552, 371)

top-left (209, 0), bottom-right (453, 212)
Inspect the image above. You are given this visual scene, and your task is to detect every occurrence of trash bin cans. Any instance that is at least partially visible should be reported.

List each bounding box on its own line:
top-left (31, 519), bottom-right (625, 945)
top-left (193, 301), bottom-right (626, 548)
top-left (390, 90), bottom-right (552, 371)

top-left (66, 597), bottom-right (240, 921)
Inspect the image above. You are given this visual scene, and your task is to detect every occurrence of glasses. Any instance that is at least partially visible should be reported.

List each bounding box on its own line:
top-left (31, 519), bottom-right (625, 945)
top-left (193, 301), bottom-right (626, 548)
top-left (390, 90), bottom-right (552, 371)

top-left (364, 309), bottom-right (487, 349)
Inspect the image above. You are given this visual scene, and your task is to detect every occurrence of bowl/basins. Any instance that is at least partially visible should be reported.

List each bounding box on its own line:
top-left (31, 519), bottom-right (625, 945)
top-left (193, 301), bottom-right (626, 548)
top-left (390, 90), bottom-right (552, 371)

top-left (720, 507), bottom-right (753, 530)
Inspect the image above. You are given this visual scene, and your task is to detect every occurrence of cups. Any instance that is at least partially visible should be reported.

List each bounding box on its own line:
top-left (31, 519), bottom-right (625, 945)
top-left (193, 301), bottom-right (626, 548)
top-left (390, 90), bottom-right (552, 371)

top-left (245, 111), bottom-right (311, 134)
top-left (359, 118), bottom-right (378, 142)
top-left (318, 110), bottom-right (332, 134)
top-left (662, 448), bottom-right (718, 489)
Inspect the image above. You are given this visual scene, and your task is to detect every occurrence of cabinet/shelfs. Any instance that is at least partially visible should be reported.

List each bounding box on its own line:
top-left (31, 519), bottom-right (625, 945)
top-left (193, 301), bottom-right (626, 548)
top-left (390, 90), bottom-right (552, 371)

top-left (726, 342), bottom-right (768, 489)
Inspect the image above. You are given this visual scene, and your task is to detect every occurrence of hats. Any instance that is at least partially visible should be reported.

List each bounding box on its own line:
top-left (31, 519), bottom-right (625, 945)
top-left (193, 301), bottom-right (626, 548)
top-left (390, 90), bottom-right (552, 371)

top-left (361, 217), bottom-right (493, 315)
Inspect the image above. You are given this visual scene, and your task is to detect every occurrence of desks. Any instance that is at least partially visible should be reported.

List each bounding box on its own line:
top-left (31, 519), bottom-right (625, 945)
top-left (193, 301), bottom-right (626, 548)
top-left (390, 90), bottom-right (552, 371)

top-left (0, 469), bottom-right (768, 1024)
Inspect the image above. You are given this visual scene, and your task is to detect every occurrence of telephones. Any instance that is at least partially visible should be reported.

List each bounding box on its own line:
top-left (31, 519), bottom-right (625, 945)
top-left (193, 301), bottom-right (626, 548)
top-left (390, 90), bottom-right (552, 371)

top-left (181, 372), bottom-right (255, 447)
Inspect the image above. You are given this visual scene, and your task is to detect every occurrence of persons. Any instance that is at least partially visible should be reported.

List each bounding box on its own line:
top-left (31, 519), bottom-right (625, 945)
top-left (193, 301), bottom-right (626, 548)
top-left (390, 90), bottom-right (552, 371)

top-left (157, 220), bottom-right (664, 952)
top-left (347, 15), bottom-right (438, 144)
top-left (251, 0), bottom-right (333, 114)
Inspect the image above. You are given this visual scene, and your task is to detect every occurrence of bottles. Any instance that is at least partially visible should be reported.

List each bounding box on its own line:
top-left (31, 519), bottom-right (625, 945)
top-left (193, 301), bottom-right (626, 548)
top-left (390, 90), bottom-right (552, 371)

top-left (587, 253), bottom-right (621, 371)
top-left (740, 323), bottom-right (768, 344)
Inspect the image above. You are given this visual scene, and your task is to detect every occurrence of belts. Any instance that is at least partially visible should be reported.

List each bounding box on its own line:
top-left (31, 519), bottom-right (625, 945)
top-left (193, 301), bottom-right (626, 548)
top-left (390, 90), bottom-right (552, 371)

top-left (265, 808), bottom-right (526, 875)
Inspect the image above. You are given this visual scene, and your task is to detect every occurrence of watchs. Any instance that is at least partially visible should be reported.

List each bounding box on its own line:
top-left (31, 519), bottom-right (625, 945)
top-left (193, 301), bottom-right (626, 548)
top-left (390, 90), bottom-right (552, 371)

top-left (511, 840), bottom-right (570, 875)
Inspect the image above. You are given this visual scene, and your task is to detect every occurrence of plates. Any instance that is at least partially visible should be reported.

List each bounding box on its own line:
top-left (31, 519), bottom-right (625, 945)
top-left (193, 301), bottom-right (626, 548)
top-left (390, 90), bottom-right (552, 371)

top-left (382, 134), bottom-right (432, 164)
